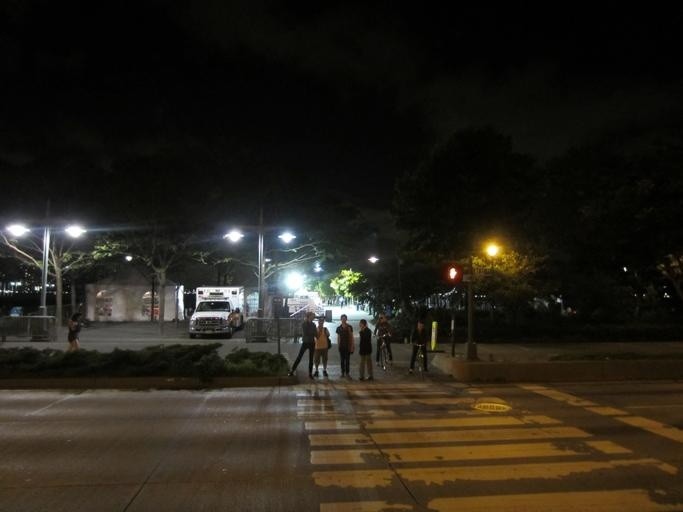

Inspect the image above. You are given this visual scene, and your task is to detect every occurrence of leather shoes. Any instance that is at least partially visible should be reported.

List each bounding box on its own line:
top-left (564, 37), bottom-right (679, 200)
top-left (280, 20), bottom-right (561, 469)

top-left (287, 370), bottom-right (373, 380)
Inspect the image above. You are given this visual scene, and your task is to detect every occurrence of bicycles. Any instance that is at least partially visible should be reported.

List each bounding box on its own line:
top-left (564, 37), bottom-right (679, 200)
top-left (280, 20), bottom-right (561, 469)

top-left (410, 343), bottom-right (427, 381)
top-left (373, 333), bottom-right (391, 371)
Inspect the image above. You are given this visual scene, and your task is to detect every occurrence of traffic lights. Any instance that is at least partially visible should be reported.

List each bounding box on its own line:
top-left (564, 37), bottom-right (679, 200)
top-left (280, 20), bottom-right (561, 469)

top-left (445, 263), bottom-right (463, 283)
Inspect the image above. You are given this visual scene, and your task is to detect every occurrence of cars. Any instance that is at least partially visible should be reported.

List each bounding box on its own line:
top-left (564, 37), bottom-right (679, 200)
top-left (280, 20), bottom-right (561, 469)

top-left (10, 306), bottom-right (23, 317)
top-left (188, 300), bottom-right (243, 339)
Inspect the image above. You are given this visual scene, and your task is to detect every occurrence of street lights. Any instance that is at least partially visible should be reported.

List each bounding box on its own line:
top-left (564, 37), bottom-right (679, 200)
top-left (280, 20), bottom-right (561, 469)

top-left (222, 230), bottom-right (296, 342)
top-left (367, 255), bottom-right (402, 301)
top-left (124, 255), bottom-right (155, 323)
top-left (6, 224), bottom-right (86, 340)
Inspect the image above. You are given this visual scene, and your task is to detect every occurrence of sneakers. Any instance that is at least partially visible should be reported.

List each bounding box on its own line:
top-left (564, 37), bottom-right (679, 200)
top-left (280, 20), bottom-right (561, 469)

top-left (408, 368), bottom-right (413, 375)
top-left (423, 369), bottom-right (430, 374)
top-left (375, 361), bottom-right (380, 368)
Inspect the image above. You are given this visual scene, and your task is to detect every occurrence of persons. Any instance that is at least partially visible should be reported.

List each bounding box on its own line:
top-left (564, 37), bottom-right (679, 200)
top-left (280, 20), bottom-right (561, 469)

top-left (287, 312), bottom-right (319, 380)
top-left (408, 320), bottom-right (429, 373)
top-left (313, 318), bottom-right (331, 377)
top-left (336, 314), bottom-right (353, 379)
top-left (373, 311), bottom-right (393, 366)
top-left (358, 319), bottom-right (374, 382)
top-left (67, 312), bottom-right (82, 352)
